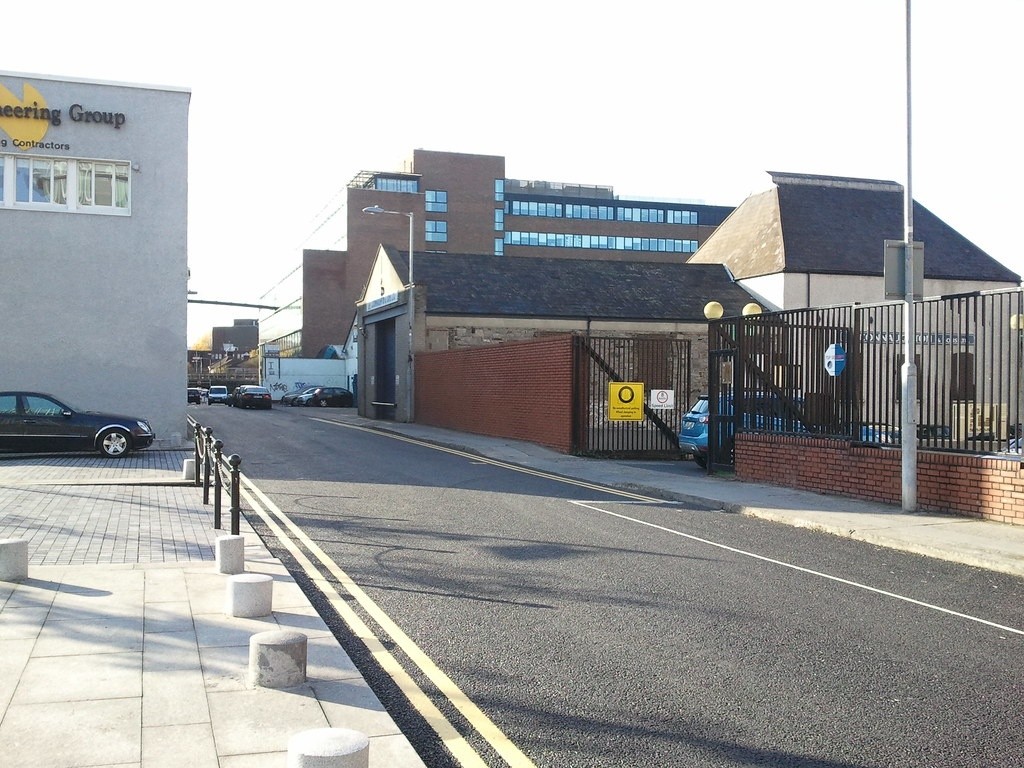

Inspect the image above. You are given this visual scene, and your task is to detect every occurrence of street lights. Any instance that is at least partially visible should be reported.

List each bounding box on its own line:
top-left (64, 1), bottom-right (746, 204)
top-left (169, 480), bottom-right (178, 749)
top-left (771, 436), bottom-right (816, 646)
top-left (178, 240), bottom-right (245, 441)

top-left (363, 205), bottom-right (414, 423)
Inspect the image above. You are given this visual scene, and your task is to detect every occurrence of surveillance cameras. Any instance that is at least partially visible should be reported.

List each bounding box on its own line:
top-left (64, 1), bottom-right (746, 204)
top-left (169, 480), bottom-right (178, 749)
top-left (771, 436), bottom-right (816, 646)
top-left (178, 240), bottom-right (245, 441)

top-left (353, 323), bottom-right (357, 327)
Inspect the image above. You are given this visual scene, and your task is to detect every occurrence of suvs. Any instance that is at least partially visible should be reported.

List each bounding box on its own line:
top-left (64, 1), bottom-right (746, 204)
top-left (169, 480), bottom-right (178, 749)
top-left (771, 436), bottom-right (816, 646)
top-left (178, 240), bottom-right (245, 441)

top-left (187, 388), bottom-right (202, 405)
top-left (206, 385), bottom-right (230, 405)
top-left (678, 388), bottom-right (894, 471)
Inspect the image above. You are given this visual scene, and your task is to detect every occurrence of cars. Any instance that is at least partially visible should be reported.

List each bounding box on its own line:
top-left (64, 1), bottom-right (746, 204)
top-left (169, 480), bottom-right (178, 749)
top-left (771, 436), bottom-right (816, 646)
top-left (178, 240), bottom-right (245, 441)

top-left (995, 422), bottom-right (1022, 454)
top-left (311, 386), bottom-right (353, 408)
top-left (0, 390), bottom-right (157, 460)
top-left (967, 431), bottom-right (1006, 442)
top-left (888, 423), bottom-right (950, 444)
top-left (238, 386), bottom-right (272, 409)
top-left (282, 385), bottom-right (315, 407)
top-left (297, 386), bottom-right (322, 407)
top-left (197, 385), bottom-right (261, 407)
